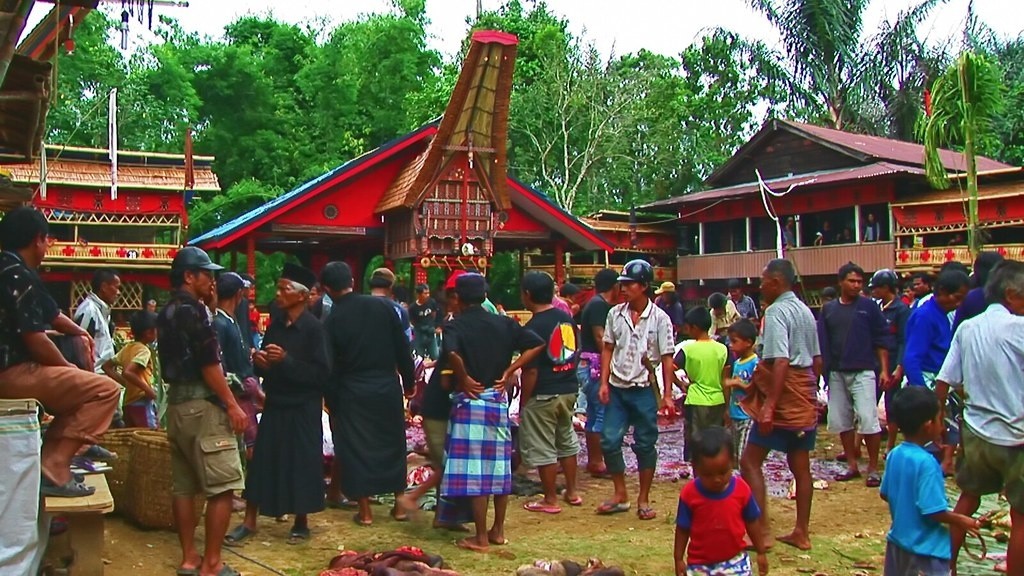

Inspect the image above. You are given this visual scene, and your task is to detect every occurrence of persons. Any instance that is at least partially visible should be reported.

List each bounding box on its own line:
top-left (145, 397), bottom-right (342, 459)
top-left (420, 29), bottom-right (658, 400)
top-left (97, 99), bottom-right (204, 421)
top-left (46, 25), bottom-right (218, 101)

top-left (1, 195), bottom-right (1024, 576)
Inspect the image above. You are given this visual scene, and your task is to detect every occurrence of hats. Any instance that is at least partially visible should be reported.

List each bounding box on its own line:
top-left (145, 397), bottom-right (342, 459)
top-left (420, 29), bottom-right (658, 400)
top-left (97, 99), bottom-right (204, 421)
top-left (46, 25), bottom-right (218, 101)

top-left (171, 244), bottom-right (899, 295)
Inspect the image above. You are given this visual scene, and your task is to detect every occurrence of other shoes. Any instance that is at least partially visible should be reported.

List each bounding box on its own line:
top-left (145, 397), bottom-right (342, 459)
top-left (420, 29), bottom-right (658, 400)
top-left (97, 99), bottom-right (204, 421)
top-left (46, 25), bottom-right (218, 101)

top-left (325, 491), bottom-right (354, 511)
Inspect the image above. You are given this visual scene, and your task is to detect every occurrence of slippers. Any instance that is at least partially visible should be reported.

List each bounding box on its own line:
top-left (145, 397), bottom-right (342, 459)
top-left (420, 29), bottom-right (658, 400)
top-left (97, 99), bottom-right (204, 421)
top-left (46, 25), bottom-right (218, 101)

top-left (594, 499), bottom-right (630, 514)
top-left (560, 488), bottom-right (582, 505)
top-left (456, 536), bottom-right (487, 549)
top-left (836, 452), bottom-right (847, 462)
top-left (488, 538), bottom-right (504, 545)
top-left (70, 445), bottom-right (118, 460)
top-left (40, 480), bottom-right (96, 498)
top-left (354, 512), bottom-right (371, 527)
top-left (214, 562), bottom-right (242, 576)
top-left (390, 508), bottom-right (410, 522)
top-left (287, 526), bottom-right (310, 545)
top-left (865, 475), bottom-right (882, 488)
top-left (523, 500), bottom-right (561, 513)
top-left (591, 468), bottom-right (613, 479)
top-left (69, 460), bottom-right (113, 475)
top-left (69, 454), bottom-right (107, 469)
top-left (835, 470), bottom-right (861, 481)
top-left (177, 555), bottom-right (205, 575)
top-left (638, 504), bottom-right (657, 521)
top-left (224, 525), bottom-right (257, 545)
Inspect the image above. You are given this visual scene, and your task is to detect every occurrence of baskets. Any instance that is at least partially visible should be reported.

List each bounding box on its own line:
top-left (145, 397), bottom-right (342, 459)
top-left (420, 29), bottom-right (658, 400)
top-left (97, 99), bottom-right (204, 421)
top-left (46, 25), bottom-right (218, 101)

top-left (123, 429), bottom-right (208, 533)
top-left (95, 426), bottom-right (151, 514)
